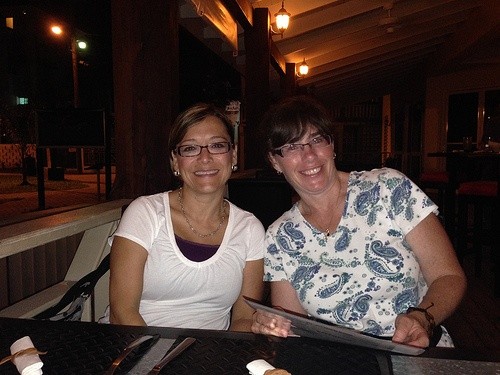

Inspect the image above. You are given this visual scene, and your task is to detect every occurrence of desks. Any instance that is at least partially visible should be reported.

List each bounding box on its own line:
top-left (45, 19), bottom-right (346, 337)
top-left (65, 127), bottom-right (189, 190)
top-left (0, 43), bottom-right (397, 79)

top-left (227, 168), bottom-right (292, 233)
top-left (427, 150), bottom-right (500, 247)
top-left (0, 316), bottom-right (500, 375)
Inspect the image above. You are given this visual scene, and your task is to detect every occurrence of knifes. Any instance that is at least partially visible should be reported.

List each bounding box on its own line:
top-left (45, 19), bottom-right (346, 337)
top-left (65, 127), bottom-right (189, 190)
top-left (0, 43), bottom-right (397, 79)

top-left (103, 335), bottom-right (154, 375)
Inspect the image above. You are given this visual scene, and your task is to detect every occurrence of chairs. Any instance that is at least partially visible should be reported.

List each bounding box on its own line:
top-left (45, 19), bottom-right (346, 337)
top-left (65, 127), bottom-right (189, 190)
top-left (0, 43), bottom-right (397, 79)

top-left (32, 204), bottom-right (130, 324)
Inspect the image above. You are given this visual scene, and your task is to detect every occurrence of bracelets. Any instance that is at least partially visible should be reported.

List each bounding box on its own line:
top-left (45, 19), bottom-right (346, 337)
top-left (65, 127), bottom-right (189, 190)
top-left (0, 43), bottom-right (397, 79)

top-left (406, 302), bottom-right (437, 341)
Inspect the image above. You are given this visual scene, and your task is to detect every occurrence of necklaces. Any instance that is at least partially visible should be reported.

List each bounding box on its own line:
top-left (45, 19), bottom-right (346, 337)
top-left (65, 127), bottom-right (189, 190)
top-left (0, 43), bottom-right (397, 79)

top-left (301, 177), bottom-right (341, 237)
top-left (179, 187), bottom-right (227, 238)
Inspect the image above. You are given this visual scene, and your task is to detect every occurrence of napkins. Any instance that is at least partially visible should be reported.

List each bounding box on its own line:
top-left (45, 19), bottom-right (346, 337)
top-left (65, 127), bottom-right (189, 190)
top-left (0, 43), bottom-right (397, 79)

top-left (0, 335), bottom-right (44, 375)
top-left (246, 359), bottom-right (291, 375)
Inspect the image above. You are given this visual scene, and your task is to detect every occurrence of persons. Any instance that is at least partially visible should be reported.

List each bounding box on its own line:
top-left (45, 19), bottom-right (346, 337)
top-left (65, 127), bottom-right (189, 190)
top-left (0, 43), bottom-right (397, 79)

top-left (107, 105), bottom-right (265, 332)
top-left (251, 102), bottom-right (467, 348)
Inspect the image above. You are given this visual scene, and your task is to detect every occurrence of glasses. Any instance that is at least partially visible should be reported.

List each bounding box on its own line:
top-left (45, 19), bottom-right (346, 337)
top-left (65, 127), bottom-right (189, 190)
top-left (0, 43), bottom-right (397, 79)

top-left (174, 141), bottom-right (234, 157)
top-left (271, 135), bottom-right (333, 158)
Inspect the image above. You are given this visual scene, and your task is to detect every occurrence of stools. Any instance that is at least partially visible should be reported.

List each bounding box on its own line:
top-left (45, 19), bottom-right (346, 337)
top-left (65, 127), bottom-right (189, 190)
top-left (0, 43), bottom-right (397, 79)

top-left (418, 171), bottom-right (449, 235)
top-left (458, 181), bottom-right (500, 298)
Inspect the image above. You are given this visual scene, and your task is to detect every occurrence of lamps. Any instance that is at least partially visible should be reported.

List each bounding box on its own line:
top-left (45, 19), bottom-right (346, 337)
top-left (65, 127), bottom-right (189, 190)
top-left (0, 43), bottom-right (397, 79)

top-left (268, 1), bottom-right (292, 40)
top-left (295, 57), bottom-right (309, 84)
